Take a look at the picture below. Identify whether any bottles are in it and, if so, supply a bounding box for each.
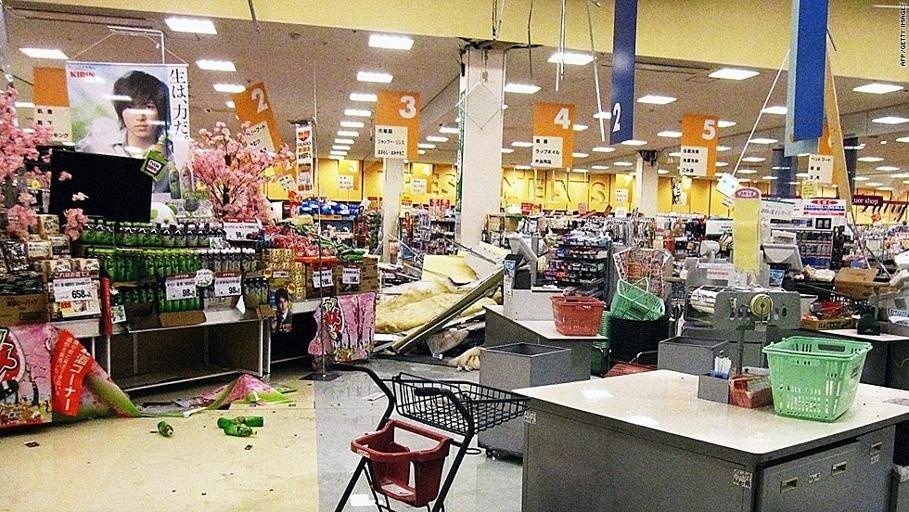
[157,421,174,436]
[216,416,264,437]
[168,161,180,199]
[179,161,193,195]
[74,217,269,315]
[144,134,167,161]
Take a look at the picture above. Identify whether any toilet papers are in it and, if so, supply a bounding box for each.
[697,354,732,402]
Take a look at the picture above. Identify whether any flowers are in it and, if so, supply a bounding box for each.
[0,54,94,250]
[179,116,296,224]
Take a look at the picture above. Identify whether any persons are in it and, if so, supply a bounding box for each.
[431,199,439,206]
[651,217,656,240]
[668,219,671,229]
[403,212,412,236]
[834,226,845,262]
[415,203,424,209]
[693,218,705,237]
[271,287,292,333]
[898,221,909,232]
[441,199,449,208]
[85,69,178,193]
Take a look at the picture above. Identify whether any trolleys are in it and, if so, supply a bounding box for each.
[321,358,524,512]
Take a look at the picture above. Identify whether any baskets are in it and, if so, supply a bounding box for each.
[588,275,679,379]
[548,295,606,337]
[760,334,874,425]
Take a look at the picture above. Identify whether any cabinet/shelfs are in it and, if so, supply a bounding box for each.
[400,201,909,310]
[0,200,381,423]
[507,364,908,511]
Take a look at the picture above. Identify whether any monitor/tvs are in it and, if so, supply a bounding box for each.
[48,150,156,224]
[762,243,804,272]
